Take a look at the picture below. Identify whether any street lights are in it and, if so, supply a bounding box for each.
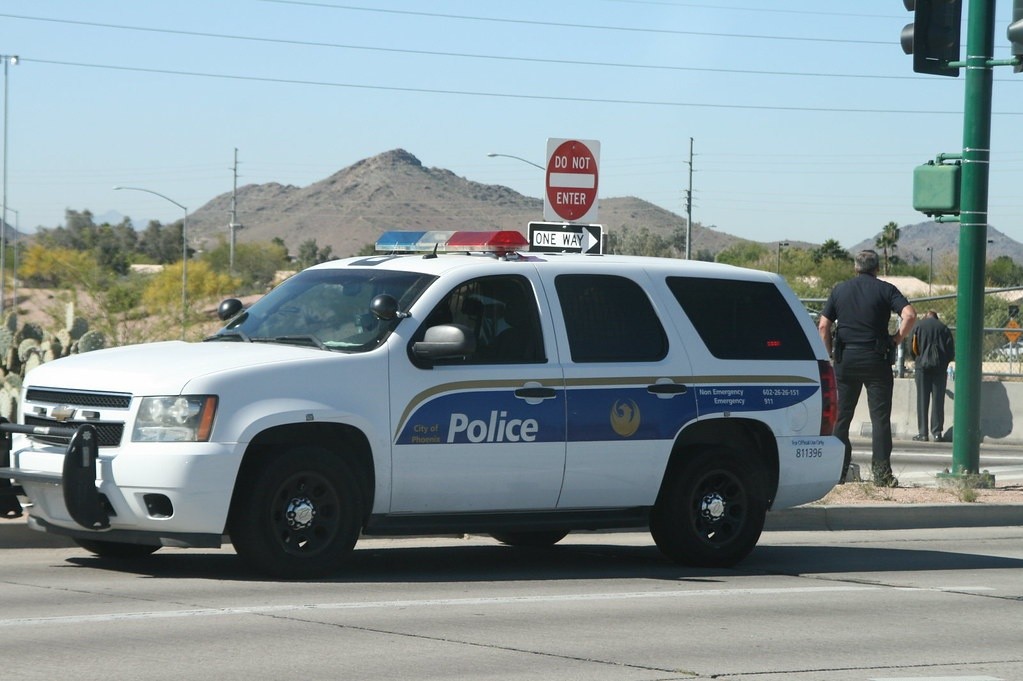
[924,247,933,298]
[113,186,187,341]
[777,241,789,276]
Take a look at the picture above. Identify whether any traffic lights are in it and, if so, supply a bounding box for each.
[542,137,601,225]
[900,0,961,77]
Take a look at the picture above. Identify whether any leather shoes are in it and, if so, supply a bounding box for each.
[934,434,944,442]
[912,434,927,441]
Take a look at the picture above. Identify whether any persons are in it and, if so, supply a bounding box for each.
[818,249,916,487]
[906,309,955,442]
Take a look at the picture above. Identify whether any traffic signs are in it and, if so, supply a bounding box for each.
[528,221,605,255]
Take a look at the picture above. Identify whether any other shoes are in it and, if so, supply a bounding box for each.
[875,474,899,487]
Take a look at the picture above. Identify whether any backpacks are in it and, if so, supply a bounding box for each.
[919,324,947,371]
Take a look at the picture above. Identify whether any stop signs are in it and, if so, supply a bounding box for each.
[7,229,852,585]
